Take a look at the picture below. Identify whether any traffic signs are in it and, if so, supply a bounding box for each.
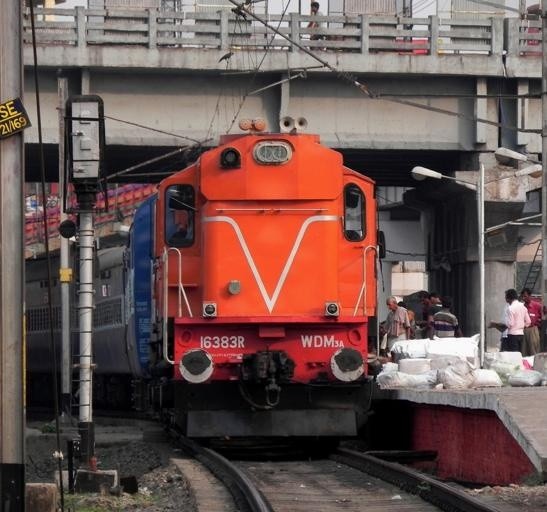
[411,164,487,370]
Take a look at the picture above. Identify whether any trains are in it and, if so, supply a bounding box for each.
[23,120,385,455]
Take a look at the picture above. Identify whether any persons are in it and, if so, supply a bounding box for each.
[378,291,464,364]
[487,288,531,355]
[518,288,542,357]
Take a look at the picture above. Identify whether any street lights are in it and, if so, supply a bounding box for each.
[493,144,544,171]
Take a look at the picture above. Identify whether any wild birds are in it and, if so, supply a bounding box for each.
[218,52,234,63]
[230,7,248,17]
